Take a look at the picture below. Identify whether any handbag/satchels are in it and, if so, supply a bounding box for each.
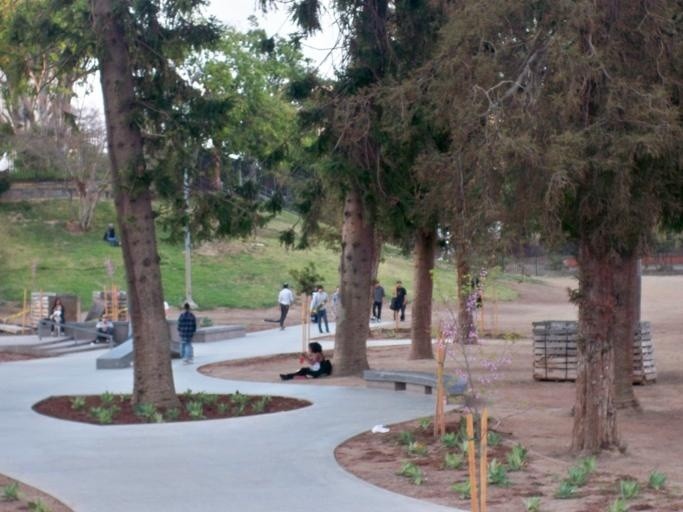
[311,312,318,323]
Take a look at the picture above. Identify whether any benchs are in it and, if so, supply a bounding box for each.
[362,368,451,393]
[194,325,247,344]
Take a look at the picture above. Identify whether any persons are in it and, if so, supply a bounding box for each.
[371,280,384,322]
[89,313,116,347]
[277,282,294,331]
[50,297,64,335]
[332,286,338,322]
[393,281,407,321]
[278,342,331,380]
[176,302,196,363]
[310,285,329,334]
[102,223,121,245]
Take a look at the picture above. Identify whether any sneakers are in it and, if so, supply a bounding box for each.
[182,360,194,365]
[279,373,293,380]
[371,316,406,322]
[280,325,329,333]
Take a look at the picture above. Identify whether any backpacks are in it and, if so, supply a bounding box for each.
[323,360,332,375]
